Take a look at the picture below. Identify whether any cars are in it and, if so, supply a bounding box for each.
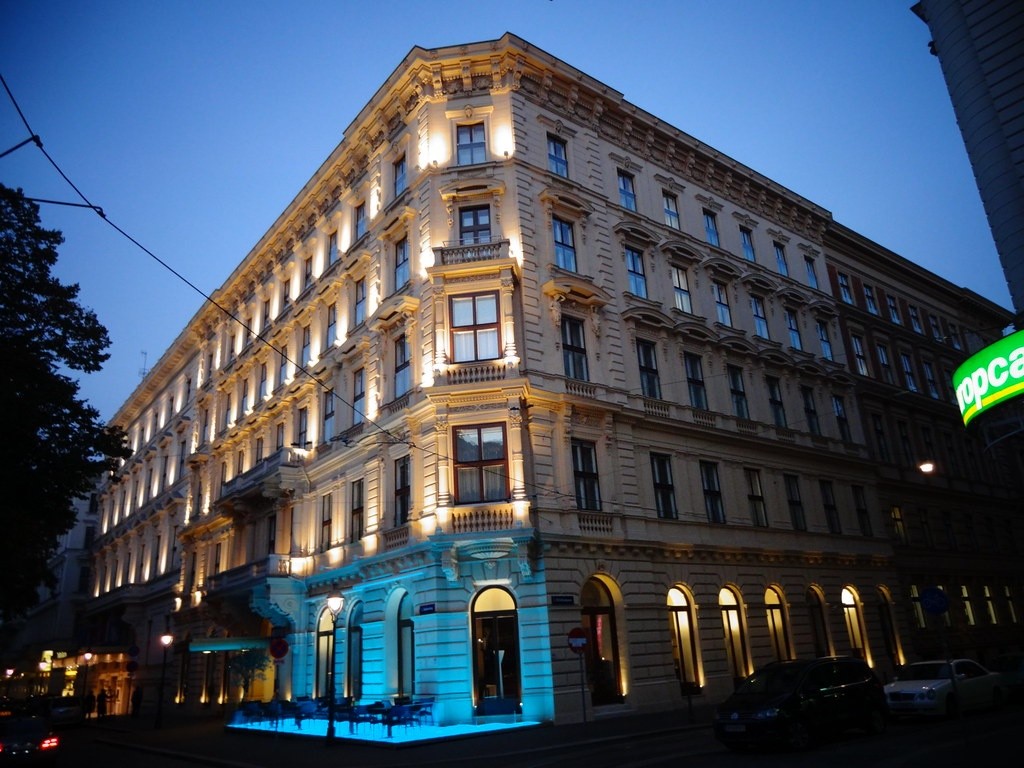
[0,713,65,768]
[711,656,889,756]
[883,656,1007,722]
[44,695,85,726]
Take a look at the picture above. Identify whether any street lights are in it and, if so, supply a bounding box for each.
[84,652,93,701]
[3,669,15,703]
[154,632,174,730]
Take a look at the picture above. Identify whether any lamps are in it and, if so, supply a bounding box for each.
[432,160,438,169]
[326,582,348,616]
[503,151,508,158]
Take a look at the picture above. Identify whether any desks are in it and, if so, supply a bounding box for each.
[371,708,388,734]
[403,703,432,726]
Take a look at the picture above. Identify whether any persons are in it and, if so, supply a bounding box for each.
[97,689,114,720]
[84,691,95,720]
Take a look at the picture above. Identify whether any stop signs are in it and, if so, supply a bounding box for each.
[567,626,588,655]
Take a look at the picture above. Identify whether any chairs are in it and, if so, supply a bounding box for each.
[235,693,437,738]
